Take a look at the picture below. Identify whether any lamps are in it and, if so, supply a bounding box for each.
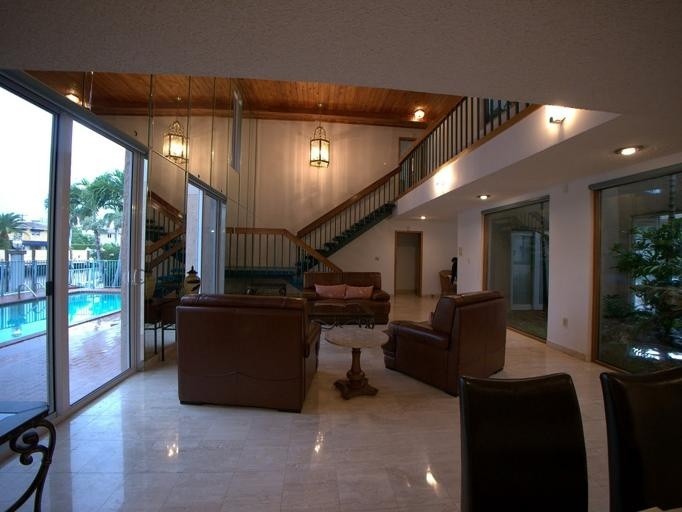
[162,96,190,166]
[308,103,331,168]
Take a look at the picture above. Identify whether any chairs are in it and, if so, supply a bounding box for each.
[455,372,592,511]
[382,288,508,398]
[175,292,324,414]
[598,364,681,509]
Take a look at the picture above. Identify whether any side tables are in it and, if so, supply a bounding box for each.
[308,304,389,400]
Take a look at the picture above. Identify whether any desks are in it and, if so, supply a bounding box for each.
[145,295,178,355]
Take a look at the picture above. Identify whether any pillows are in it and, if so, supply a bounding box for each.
[314,282,374,300]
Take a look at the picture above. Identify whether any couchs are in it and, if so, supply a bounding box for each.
[302,271,391,325]
